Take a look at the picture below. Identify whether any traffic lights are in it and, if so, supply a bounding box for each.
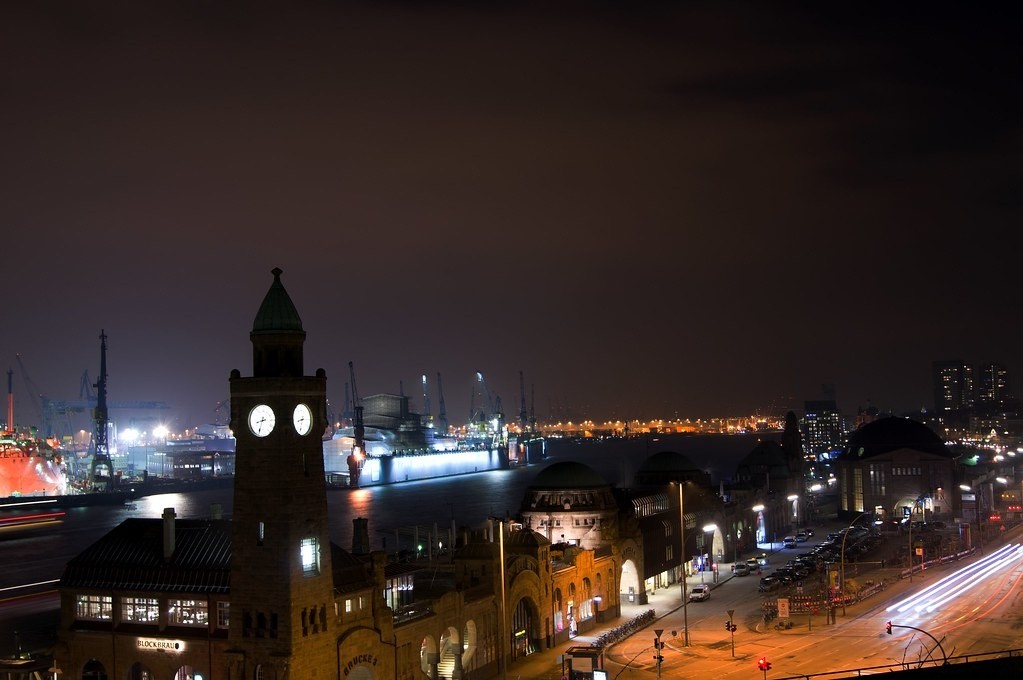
[766,661,772,671]
[654,638,657,648]
[653,656,656,659]
[659,656,664,662]
[758,665,763,671]
[660,642,664,650]
[886,620,892,634]
[732,624,737,632]
[882,559,886,568]
[724,621,731,631]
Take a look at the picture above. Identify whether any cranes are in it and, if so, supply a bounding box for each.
[340,359,538,445]
[13,326,172,494]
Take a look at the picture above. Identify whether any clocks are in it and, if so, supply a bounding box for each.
[247,404,276,438]
[292,402,314,438]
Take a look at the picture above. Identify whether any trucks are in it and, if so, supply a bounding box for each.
[689,585,712,602]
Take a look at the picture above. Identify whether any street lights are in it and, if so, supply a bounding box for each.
[907,486,943,582]
[841,511,873,617]
[759,657,767,680]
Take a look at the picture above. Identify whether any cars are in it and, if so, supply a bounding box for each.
[746,558,759,571]
[733,561,751,576]
[755,554,770,565]
[757,518,946,592]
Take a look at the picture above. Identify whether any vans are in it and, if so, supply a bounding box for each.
[783,536,797,549]
